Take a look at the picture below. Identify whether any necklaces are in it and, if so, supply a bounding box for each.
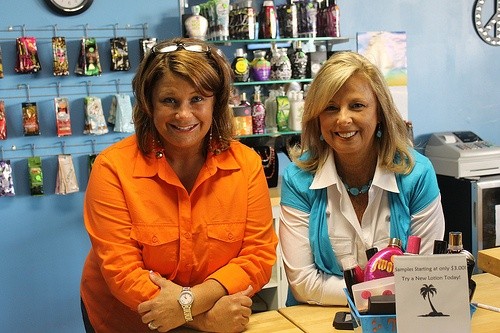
[340,177,372,196]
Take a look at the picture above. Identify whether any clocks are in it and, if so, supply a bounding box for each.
[43,0,94,15]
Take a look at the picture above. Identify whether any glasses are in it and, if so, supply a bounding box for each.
[140,40,221,80]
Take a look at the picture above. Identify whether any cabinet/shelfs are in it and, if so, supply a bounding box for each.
[180,0,352,138]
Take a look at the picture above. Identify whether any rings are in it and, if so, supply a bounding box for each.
[148,322,158,329]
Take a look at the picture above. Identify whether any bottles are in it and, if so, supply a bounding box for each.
[185,0,342,135]
[342,230,476,307]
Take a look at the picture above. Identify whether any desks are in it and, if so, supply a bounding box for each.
[238,273,500,333]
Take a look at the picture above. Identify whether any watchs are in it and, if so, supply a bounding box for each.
[178,286,194,323]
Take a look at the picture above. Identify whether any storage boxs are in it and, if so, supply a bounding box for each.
[341,285,477,333]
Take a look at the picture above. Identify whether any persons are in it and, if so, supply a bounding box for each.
[80,37,279,333]
[278,51,446,307]
